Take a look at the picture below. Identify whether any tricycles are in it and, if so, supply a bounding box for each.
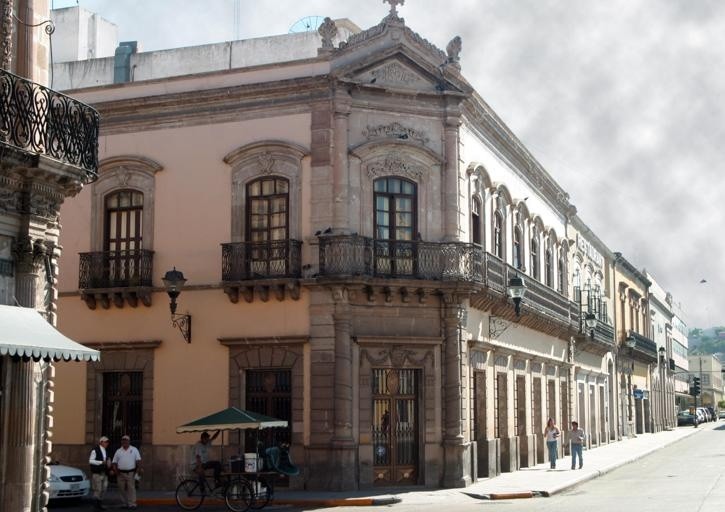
[175,406,289,512]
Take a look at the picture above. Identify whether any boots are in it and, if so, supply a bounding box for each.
[92,499,138,512]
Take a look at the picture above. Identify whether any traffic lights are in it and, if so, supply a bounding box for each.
[688,376,700,396]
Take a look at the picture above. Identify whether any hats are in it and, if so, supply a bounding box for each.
[122,435,131,440]
[100,436,109,442]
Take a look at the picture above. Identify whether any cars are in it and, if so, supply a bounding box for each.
[677,407,724,426]
[47,461,92,499]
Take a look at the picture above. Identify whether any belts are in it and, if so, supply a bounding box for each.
[119,468,136,473]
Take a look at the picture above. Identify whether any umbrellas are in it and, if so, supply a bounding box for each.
[176,406,288,474]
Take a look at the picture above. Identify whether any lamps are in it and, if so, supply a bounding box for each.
[488,273,528,339]
[658,347,665,362]
[625,332,636,352]
[585,310,598,341]
[162,267,192,344]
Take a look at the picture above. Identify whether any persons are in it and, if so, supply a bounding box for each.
[112,436,142,509]
[191,429,221,497]
[89,436,114,508]
[544,418,560,469]
[564,421,585,470]
